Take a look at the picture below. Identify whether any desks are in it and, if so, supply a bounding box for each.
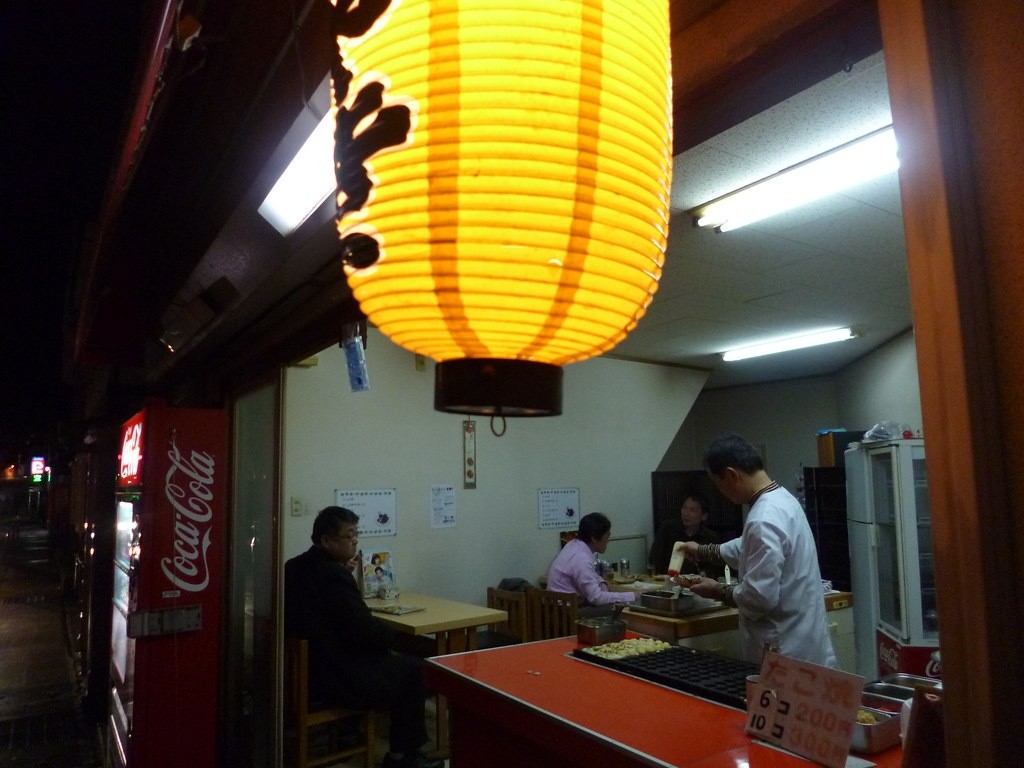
[608,573,669,594]
[364,591,509,761]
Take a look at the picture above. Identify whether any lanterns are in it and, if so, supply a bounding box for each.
[327,0,672,419]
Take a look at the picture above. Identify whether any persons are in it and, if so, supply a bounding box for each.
[282,505,437,768]
[645,488,723,584]
[679,433,839,672]
[545,511,636,619]
[364,552,396,598]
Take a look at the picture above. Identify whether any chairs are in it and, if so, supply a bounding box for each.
[256,615,376,768]
[476,586,579,650]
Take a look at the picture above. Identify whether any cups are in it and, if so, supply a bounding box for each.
[604,568,614,583]
[647,565,656,578]
[746,675,760,715]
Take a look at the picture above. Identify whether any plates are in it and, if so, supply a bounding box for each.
[613,579,635,584]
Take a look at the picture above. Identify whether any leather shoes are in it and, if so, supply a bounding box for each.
[381,751,442,768]
[337,732,365,763]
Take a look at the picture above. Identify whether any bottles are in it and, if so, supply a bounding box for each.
[667,541,686,577]
[718,576,738,586]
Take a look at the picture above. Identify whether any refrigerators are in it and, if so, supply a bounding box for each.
[105,407,230,768]
[867,439,942,679]
[844,447,878,685]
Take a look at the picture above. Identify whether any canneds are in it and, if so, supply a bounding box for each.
[621,559,630,576]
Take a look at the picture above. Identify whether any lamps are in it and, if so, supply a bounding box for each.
[720,324,865,362]
[688,125,898,234]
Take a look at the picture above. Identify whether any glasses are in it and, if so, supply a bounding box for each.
[338,532,359,540]
